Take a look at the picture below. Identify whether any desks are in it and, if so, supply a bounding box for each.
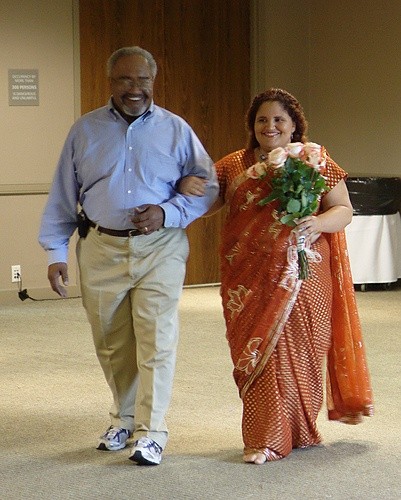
[344,212,401,291]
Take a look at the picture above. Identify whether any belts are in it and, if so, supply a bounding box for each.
[87,217,143,240]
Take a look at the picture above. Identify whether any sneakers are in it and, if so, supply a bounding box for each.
[95,425,133,452]
[127,437,162,465]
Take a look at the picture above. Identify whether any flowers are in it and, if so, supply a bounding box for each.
[244,142,330,293]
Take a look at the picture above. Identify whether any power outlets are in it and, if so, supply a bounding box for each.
[11,265,22,283]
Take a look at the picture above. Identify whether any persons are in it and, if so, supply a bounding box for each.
[178,89,374,464]
[39,47,219,464]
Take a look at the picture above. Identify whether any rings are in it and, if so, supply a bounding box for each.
[145,227,148,232]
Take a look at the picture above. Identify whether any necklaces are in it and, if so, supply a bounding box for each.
[257,147,269,162]
